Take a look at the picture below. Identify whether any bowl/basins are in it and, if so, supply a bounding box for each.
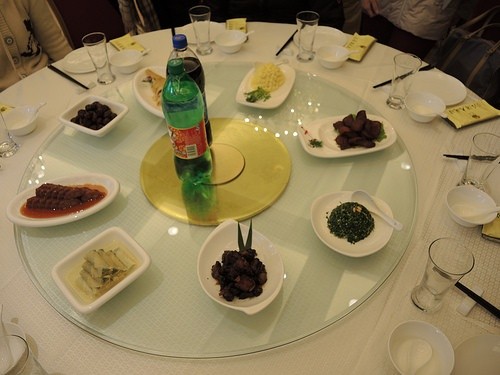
[309,191,394,258]
[51,226,152,315]
[0,20,500,375]
[58,93,129,137]
[387,320,455,374]
[196,218,284,316]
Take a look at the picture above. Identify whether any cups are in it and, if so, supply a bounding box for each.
[188,4,213,55]
[410,237,475,312]
[0,334,50,375]
[0,113,21,157]
[457,130,500,189]
[387,52,421,110]
[84,30,116,85]
[295,11,320,62]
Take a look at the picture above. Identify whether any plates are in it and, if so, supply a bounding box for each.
[297,115,397,158]
[0,317,27,375]
[132,65,178,120]
[235,62,297,109]
[6,175,120,227]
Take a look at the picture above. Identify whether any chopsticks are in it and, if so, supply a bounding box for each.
[432,266,500,322]
[431,266,500,319]
[172,27,178,57]
[443,154,496,161]
[276,23,305,56]
[373,64,434,88]
[47,65,90,90]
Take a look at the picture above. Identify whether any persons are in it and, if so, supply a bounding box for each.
[0,0,74,94]
[358,0,461,60]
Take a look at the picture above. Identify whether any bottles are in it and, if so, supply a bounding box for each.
[167,36,212,147]
[162,61,214,185]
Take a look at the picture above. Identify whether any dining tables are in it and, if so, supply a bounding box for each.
[0,22,500,375]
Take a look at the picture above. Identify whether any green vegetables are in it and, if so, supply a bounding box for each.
[326,202,375,243]
[242,87,270,103]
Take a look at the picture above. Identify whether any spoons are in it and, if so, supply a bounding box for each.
[403,338,432,375]
[456,205,500,219]
